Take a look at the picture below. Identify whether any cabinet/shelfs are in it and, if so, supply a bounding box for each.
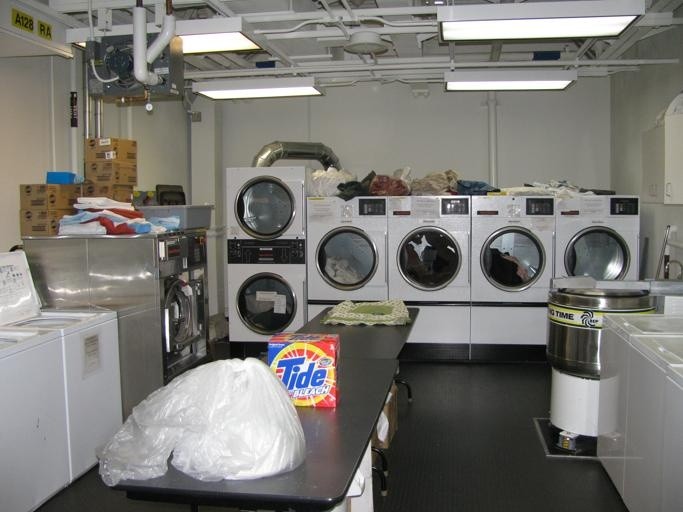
[642,112,683,205]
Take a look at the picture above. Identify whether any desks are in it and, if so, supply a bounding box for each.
[295,307,419,475]
[111,357,400,512]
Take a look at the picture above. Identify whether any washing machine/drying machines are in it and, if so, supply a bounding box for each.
[596,314,683,512]
[227,239,308,342]
[555,195,641,281]
[388,195,471,361]
[226,167,306,241]
[20,230,209,376]
[471,195,555,361]
[0,250,124,512]
[307,196,389,322]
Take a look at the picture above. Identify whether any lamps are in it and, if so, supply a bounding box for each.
[435,1,646,44]
[191,74,325,101]
[444,67,578,90]
[175,16,268,55]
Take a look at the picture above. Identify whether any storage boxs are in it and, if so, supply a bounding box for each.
[370,382,400,450]
[135,204,214,230]
[19,137,136,237]
